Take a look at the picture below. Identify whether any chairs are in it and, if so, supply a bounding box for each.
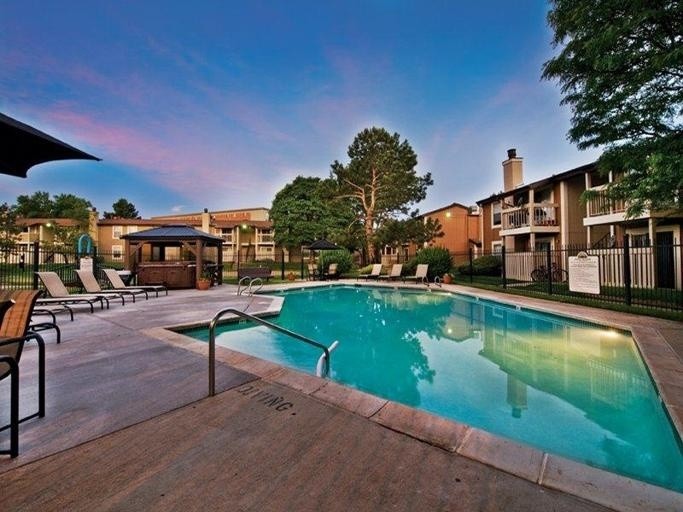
[401,263,429,284]
[377,264,403,283]
[0,261,169,461]
[356,263,382,282]
[306,263,321,280]
[323,262,341,281]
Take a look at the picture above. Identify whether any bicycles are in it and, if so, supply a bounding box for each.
[530,262,568,284]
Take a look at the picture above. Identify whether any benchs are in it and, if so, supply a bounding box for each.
[236,266,273,282]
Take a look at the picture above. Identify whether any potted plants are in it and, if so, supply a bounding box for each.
[196,272,213,290]
[442,271,454,285]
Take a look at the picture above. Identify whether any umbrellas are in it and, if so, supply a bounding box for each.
[0,102,104,181]
[302,239,343,272]
